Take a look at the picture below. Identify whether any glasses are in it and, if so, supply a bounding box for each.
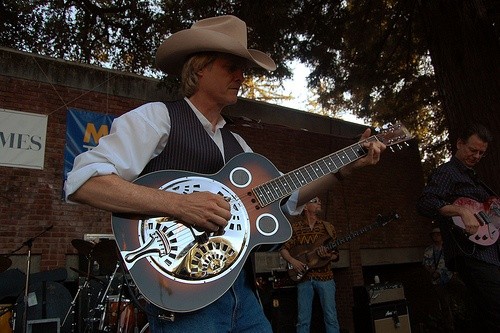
[309,198,322,203]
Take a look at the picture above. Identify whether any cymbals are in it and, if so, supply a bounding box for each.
[72,237,94,259]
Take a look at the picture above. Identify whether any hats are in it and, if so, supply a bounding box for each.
[155,15,277,72]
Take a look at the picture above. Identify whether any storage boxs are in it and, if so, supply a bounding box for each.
[368,284,407,305]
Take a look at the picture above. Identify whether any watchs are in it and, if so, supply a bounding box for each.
[331,168,345,182]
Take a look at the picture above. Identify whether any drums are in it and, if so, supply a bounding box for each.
[106,296,151,333]
[17,280,74,333]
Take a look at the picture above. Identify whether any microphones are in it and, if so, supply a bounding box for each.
[393,310,400,328]
[0,302,21,317]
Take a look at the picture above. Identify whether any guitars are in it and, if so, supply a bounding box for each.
[284,213,401,282]
[450,187,500,246]
[111,121,412,321]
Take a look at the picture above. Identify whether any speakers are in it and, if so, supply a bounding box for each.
[353,299,412,333]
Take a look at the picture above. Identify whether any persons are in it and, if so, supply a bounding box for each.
[278,196,340,333]
[63,15,387,333]
[416,123,500,333]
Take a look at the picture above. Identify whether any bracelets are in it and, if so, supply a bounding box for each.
[329,254,332,259]
